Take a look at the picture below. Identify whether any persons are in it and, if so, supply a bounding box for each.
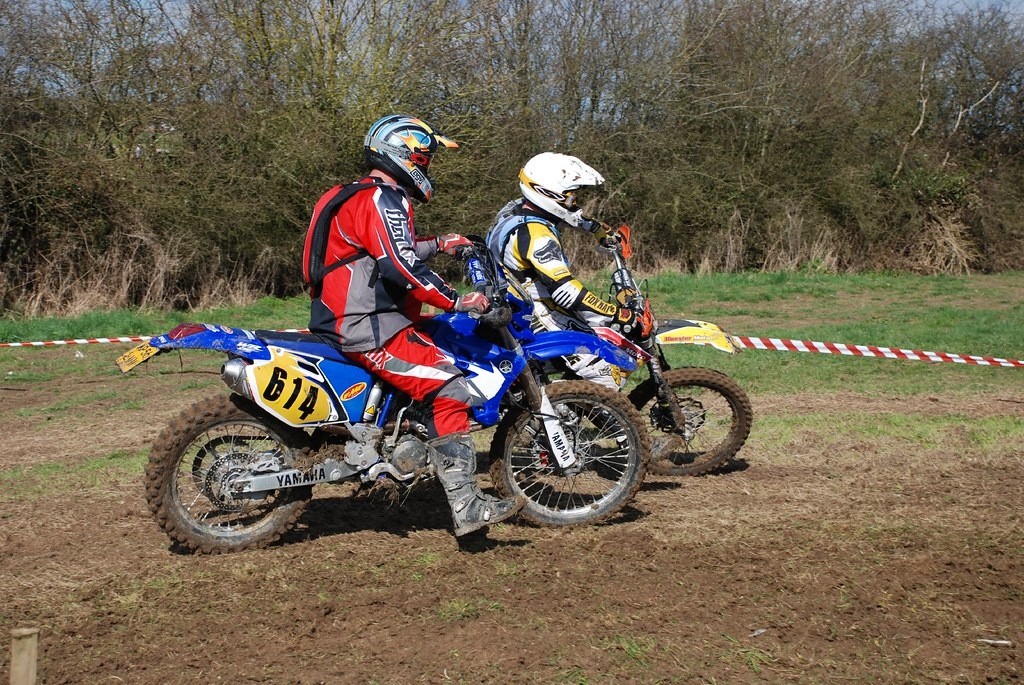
[302,115,525,541]
[484,152,654,474]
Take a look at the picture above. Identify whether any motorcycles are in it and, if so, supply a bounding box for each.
[498,223,754,481]
[114,235,651,556]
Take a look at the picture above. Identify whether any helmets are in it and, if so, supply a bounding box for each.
[517,151,605,228]
[364,114,459,203]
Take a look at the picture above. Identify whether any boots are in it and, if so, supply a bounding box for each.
[427,432,524,538]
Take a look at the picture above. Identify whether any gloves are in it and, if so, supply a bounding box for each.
[457,291,490,315]
[616,306,639,329]
[436,233,473,255]
[595,221,622,248]
